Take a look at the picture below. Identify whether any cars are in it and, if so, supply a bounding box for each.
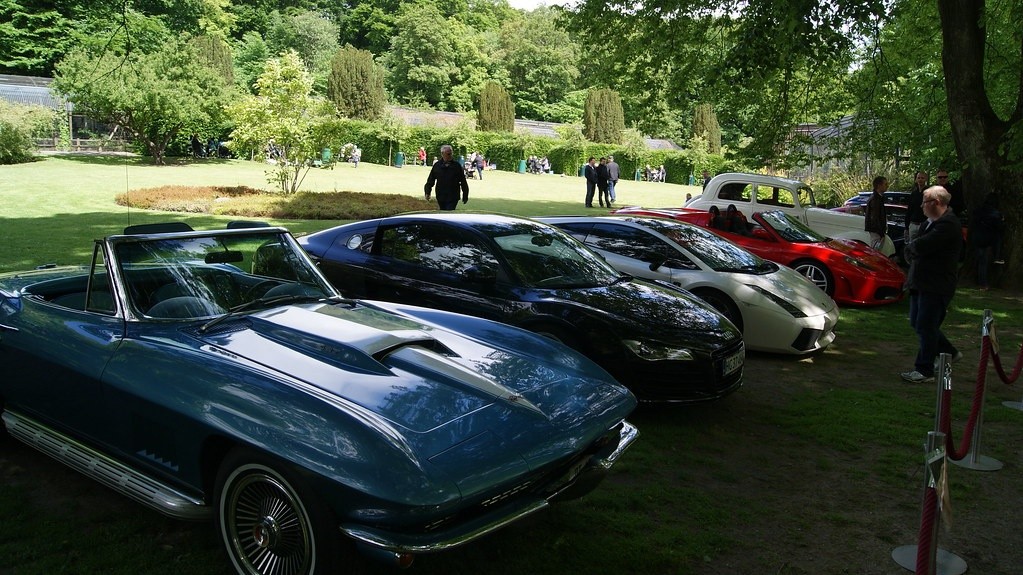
[829,191,913,266]
[682,172,897,263]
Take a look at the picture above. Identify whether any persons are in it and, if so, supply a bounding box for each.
[645,164,651,177]
[686,193,692,202]
[351,144,361,167]
[527,154,550,173]
[900,185,963,384]
[864,176,888,249]
[703,171,711,193]
[458,151,483,179]
[595,158,611,208]
[585,157,597,207]
[424,145,469,210]
[660,165,666,182]
[433,156,438,166]
[606,156,621,203]
[957,192,1003,290]
[936,170,962,216]
[419,147,427,166]
[190,134,215,159]
[904,171,929,230]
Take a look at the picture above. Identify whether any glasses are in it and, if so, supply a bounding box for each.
[937,176,948,179]
[917,177,924,179]
[922,198,939,204]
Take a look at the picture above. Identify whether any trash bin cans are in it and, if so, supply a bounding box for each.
[518,160,526,173]
[637,169,641,181]
[689,175,694,186]
[395,152,403,168]
[457,155,464,168]
[580,165,585,176]
[322,148,330,164]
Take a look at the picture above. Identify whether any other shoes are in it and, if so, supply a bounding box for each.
[586,203,611,208]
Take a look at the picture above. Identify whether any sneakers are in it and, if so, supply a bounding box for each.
[935,347,964,373]
[901,370,935,384]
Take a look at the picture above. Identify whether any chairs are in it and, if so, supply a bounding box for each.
[40,276,234,317]
[710,205,752,237]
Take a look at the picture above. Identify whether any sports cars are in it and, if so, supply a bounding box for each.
[490,213,840,360]
[0,221,644,575]
[251,209,747,421]
[610,202,909,310]
